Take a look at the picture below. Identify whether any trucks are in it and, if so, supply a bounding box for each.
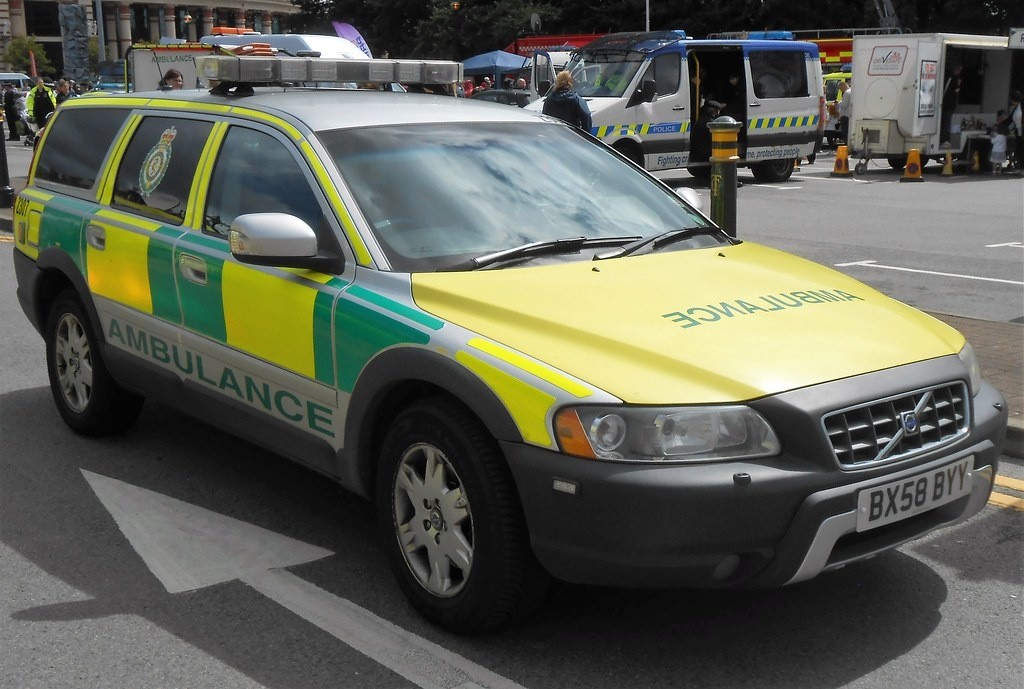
[845,27,1024,168]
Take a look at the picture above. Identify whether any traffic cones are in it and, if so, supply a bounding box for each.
[829,145,854,179]
[899,148,927,183]
[969,148,981,174]
[941,148,958,176]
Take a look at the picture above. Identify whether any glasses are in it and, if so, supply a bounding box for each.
[173,78,183,83]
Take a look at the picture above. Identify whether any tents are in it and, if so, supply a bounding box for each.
[461,49,532,75]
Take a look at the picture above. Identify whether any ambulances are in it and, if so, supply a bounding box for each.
[795,38,854,73]
[818,62,853,147]
[87,26,409,96]
[13,49,1011,644]
[517,31,829,180]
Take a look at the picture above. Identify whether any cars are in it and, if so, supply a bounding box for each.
[0,72,37,92]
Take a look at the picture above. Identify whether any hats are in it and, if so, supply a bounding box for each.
[707,101,726,109]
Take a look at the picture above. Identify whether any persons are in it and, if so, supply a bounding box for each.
[450,75,530,108]
[687,56,798,187]
[838,82,852,146]
[990,90,1024,176]
[814,85,839,157]
[356,81,448,96]
[157,69,184,91]
[380,49,389,59]
[542,70,592,133]
[796,153,816,165]
[941,73,963,148]
[0,78,95,194]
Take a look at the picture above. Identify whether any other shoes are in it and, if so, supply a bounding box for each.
[940,140,953,148]
[5,135,20,141]
[809,159,815,164]
[992,171,1001,177]
[830,151,837,155]
